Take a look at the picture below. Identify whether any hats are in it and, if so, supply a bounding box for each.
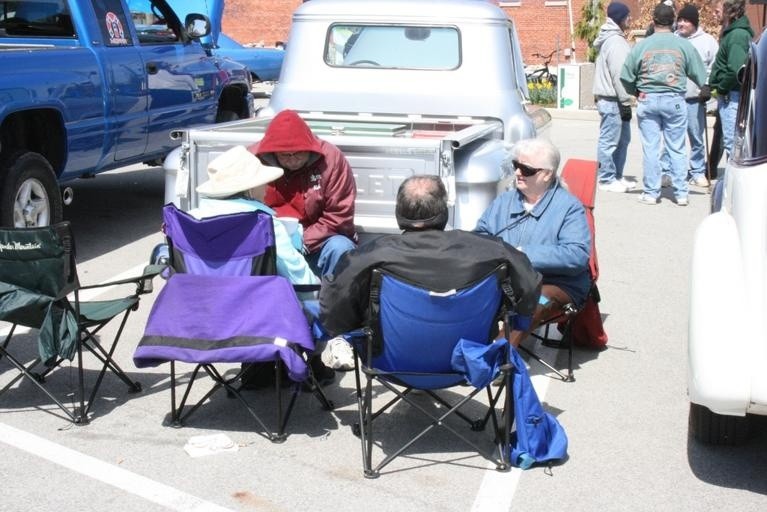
[676,5,699,31]
[649,0,675,26]
[195,145,285,198]
[607,1,630,24]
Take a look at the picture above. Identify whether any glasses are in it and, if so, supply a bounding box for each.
[512,159,553,176]
[276,151,309,161]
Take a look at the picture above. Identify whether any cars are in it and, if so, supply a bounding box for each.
[129,0,285,85]
[682,22,767,457]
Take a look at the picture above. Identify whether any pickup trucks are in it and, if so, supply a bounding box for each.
[0,0,259,235]
[158,1,553,244]
[38,84,152,150]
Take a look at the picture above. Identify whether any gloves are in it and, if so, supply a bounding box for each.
[716,86,730,95]
[619,104,632,121]
[699,84,713,103]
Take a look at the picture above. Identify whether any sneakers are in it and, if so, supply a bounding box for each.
[598,178,627,193]
[638,191,658,204]
[617,176,636,189]
[239,352,336,392]
[694,174,710,188]
[327,336,357,373]
[675,196,689,205]
[661,174,674,188]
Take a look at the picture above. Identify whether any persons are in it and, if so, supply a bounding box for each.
[620,4,708,206]
[170,143,335,392]
[255,109,357,298]
[658,5,720,189]
[646,1,679,37]
[593,1,635,186]
[708,0,754,162]
[318,175,542,357]
[473,138,593,386]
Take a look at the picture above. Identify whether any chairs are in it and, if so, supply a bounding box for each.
[0,220,164,426]
[518,158,601,382]
[341,262,521,479]
[162,202,335,442]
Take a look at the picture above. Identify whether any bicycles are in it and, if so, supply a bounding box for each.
[524,49,561,86]
